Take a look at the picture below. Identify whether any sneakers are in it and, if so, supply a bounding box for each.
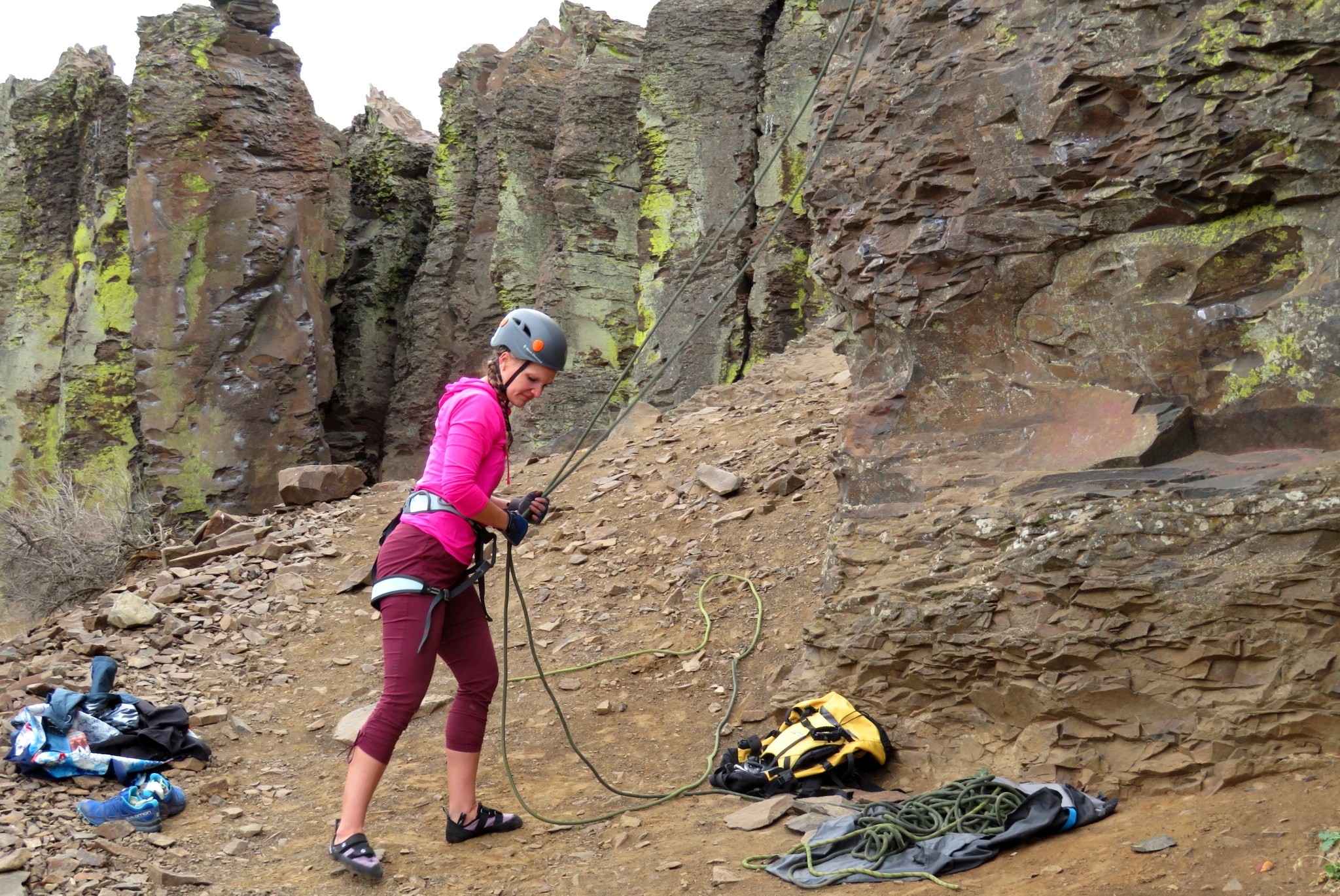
[77,785,162,834]
[138,773,185,818]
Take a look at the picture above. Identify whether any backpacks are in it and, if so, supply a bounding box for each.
[708,690,899,803]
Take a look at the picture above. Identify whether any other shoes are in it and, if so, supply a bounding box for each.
[329,819,384,879]
[442,803,522,842]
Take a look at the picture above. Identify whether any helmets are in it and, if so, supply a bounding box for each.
[490,308,568,371]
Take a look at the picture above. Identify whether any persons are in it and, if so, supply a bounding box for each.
[330,309,567,881]
